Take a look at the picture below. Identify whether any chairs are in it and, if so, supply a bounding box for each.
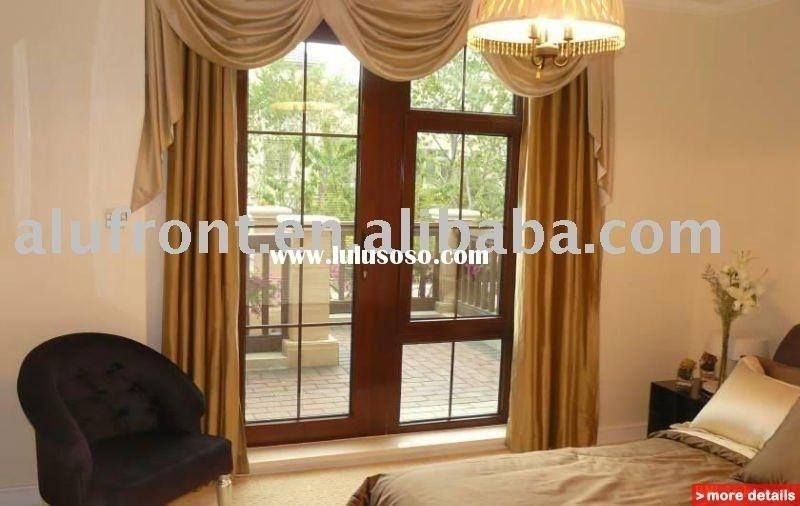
[17,333,234,506]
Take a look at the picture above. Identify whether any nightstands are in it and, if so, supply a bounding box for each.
[647,378,727,435]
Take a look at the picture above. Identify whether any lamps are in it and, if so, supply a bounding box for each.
[466,0,626,81]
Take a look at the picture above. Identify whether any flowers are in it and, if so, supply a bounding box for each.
[701,248,769,335]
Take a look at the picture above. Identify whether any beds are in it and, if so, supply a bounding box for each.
[346,323,799,506]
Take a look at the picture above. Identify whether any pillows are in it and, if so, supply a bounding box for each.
[651,354,800,484]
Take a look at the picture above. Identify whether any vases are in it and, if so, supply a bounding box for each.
[678,357,697,382]
[718,336,729,389]
[699,351,718,380]
